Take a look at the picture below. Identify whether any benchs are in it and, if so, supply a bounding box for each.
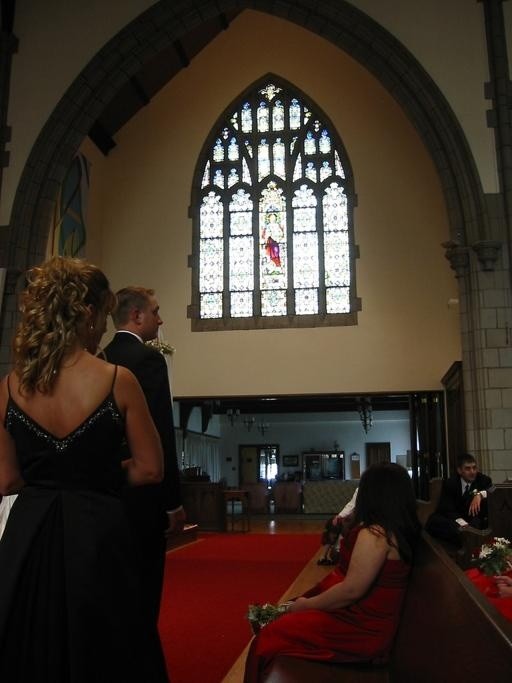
[251,527,511,683]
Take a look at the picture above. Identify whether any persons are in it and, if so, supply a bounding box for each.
[241,459,429,681]
[95,284,186,626]
[261,213,283,272]
[1,254,169,683]
[315,481,361,567]
[422,451,495,549]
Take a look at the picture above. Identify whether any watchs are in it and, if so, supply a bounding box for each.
[476,493,483,499]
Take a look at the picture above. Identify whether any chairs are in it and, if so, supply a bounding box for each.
[242,479,358,515]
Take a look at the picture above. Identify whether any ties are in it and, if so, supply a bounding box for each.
[461,483,470,496]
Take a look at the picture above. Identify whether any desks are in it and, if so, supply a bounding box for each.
[221,487,252,534]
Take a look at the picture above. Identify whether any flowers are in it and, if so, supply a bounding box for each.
[246,599,288,636]
[467,536,511,579]
